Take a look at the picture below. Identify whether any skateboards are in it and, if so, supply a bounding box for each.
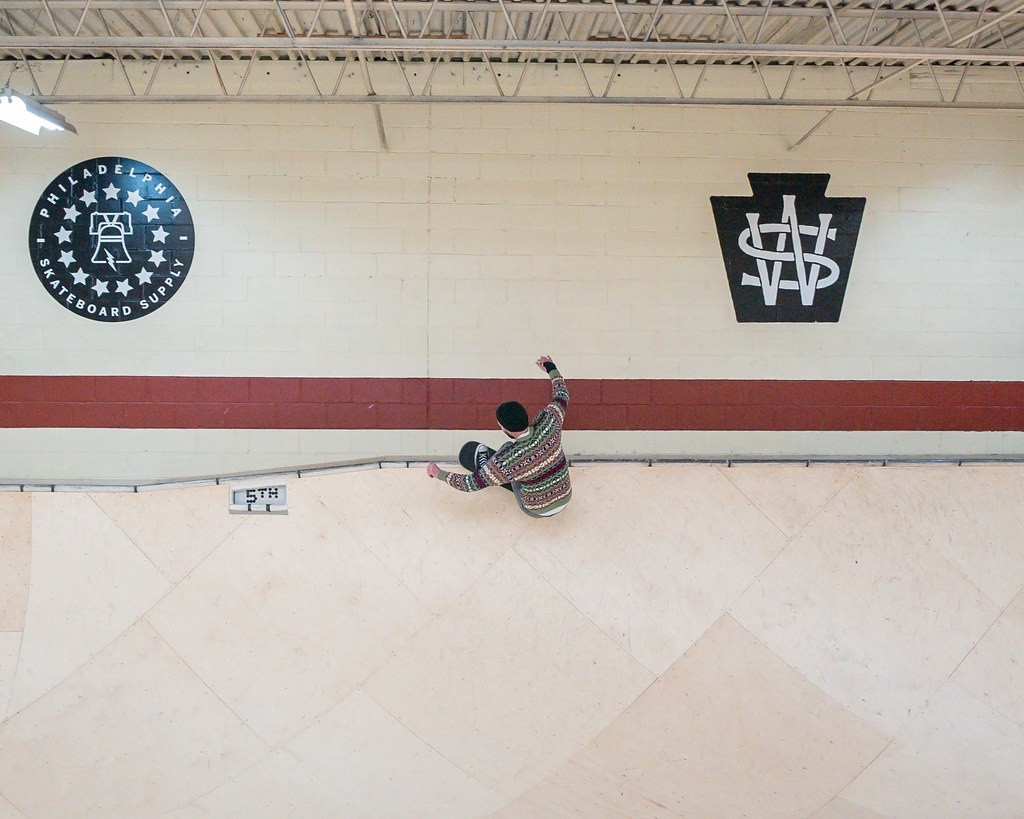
[457,440,514,493]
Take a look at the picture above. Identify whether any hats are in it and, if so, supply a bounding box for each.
[496,401,530,431]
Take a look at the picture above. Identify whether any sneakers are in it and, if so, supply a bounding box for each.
[474,445,490,469]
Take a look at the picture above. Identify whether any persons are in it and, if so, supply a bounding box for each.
[428,355,572,518]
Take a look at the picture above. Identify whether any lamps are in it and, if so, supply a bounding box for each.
[0,6,79,136]
